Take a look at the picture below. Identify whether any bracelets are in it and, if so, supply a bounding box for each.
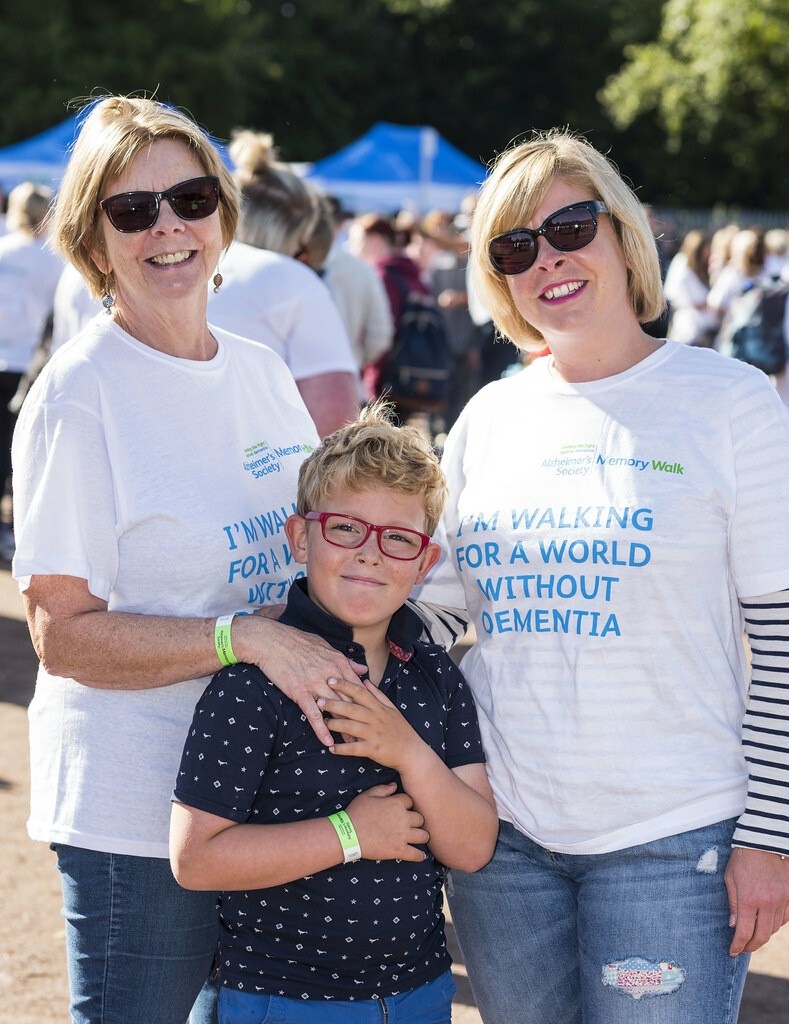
[329,808,364,865]
[215,609,246,668]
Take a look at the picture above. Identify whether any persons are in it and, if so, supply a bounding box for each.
[245,138,789,1023]
[48,219,363,446]
[0,179,63,525]
[12,98,390,1023]
[168,417,500,1024]
[212,132,787,459]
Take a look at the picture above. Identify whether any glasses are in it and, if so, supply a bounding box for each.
[485,199,610,275]
[93,175,222,234]
[305,509,434,562]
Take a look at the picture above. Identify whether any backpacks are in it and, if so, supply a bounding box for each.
[372,264,456,415]
[732,284,789,375]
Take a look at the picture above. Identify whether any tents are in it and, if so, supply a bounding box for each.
[0,92,265,212]
[284,123,511,227]
